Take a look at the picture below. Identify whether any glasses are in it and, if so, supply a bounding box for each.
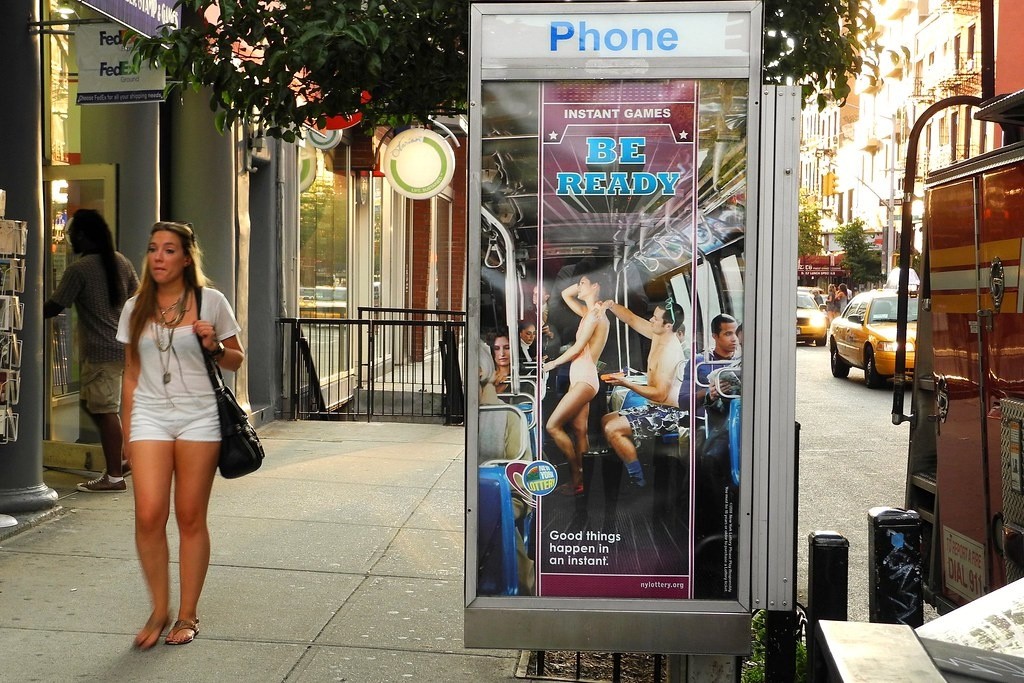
[65,229,75,236]
[175,221,197,248]
[666,296,676,326]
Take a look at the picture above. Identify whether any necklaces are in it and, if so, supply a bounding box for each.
[151,291,193,382]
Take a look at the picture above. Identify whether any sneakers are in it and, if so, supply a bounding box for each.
[76,468,127,492]
[122,460,132,477]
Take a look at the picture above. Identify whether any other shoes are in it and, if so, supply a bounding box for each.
[554,483,584,495]
[612,478,647,499]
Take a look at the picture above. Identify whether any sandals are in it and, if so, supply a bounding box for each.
[134,611,171,648]
[165,617,200,644]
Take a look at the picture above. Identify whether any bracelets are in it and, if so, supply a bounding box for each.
[609,300,614,309]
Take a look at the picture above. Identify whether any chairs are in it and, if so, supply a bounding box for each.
[476,359,741,594]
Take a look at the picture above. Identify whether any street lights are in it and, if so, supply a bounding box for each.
[820,93,895,278]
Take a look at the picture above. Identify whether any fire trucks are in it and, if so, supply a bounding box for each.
[892,90,1024,618]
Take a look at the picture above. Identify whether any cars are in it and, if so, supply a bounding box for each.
[796,291,828,346]
[828,289,919,389]
[797,286,824,296]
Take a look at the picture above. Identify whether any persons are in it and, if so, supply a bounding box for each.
[605,258,650,372]
[43,209,140,492]
[543,271,610,496]
[835,283,848,314]
[678,313,743,473]
[115,221,246,650]
[548,257,597,309]
[532,282,561,363]
[812,289,824,306]
[596,299,691,500]
[478,339,533,520]
[519,321,537,362]
[486,324,510,400]
[826,283,835,322]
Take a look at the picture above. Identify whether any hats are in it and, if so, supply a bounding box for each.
[812,289,818,293]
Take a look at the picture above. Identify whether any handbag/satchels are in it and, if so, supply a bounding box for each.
[194,285,265,479]
